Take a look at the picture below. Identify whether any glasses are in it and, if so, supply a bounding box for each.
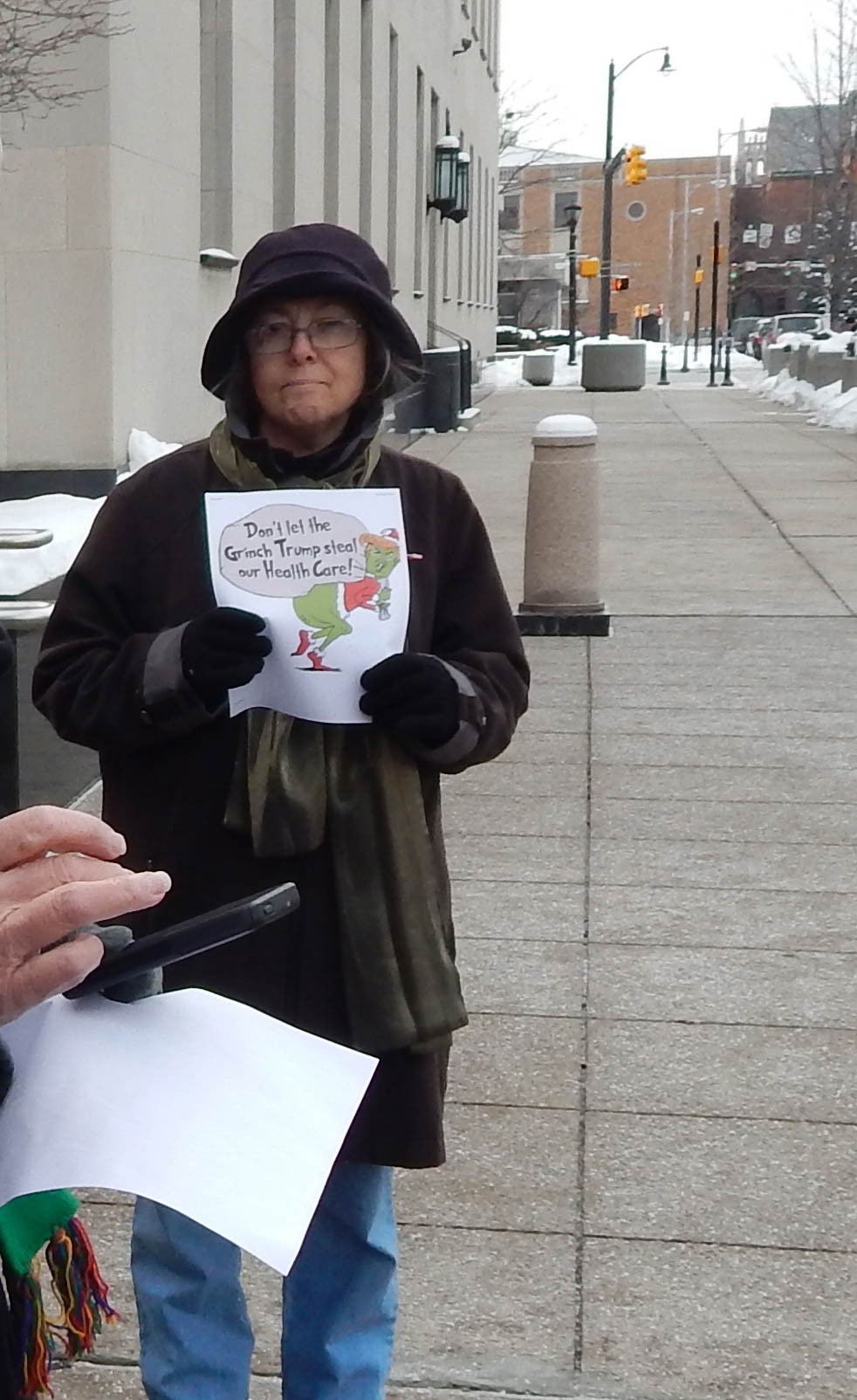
[243,315,362,357]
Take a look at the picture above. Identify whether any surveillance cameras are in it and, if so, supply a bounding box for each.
[460,38,471,52]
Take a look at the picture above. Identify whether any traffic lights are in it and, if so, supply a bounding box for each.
[615,276,629,290]
[694,270,704,285]
[623,148,647,187]
[712,243,726,266]
[578,257,599,276]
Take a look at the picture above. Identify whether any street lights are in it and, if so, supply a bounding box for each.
[599,45,672,341]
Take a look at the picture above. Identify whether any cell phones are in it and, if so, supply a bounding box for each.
[58,880,302,1000]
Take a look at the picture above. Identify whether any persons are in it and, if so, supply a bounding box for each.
[2,807,174,1399]
[33,228,530,1400]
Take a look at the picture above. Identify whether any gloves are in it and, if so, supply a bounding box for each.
[361,650,460,753]
[181,606,272,704]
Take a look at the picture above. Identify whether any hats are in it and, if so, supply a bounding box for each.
[201,224,423,403]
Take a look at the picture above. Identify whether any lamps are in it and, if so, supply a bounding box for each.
[440,152,470,226]
[426,135,461,217]
